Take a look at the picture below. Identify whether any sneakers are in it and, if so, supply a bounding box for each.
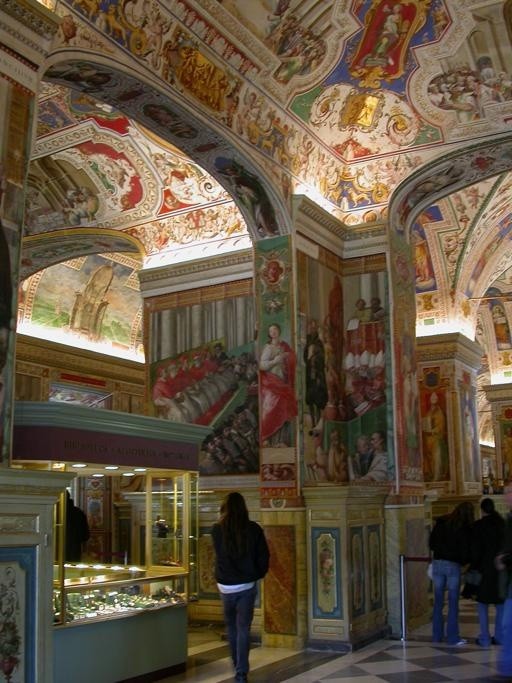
[234,671,247,683]
[456,638,468,645]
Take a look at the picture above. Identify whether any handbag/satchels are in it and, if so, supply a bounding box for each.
[463,564,483,586]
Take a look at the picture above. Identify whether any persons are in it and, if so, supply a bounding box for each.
[208,489,271,681]
[428,500,475,646]
[460,496,509,647]
[493,506,512,681]
[63,486,93,562]
